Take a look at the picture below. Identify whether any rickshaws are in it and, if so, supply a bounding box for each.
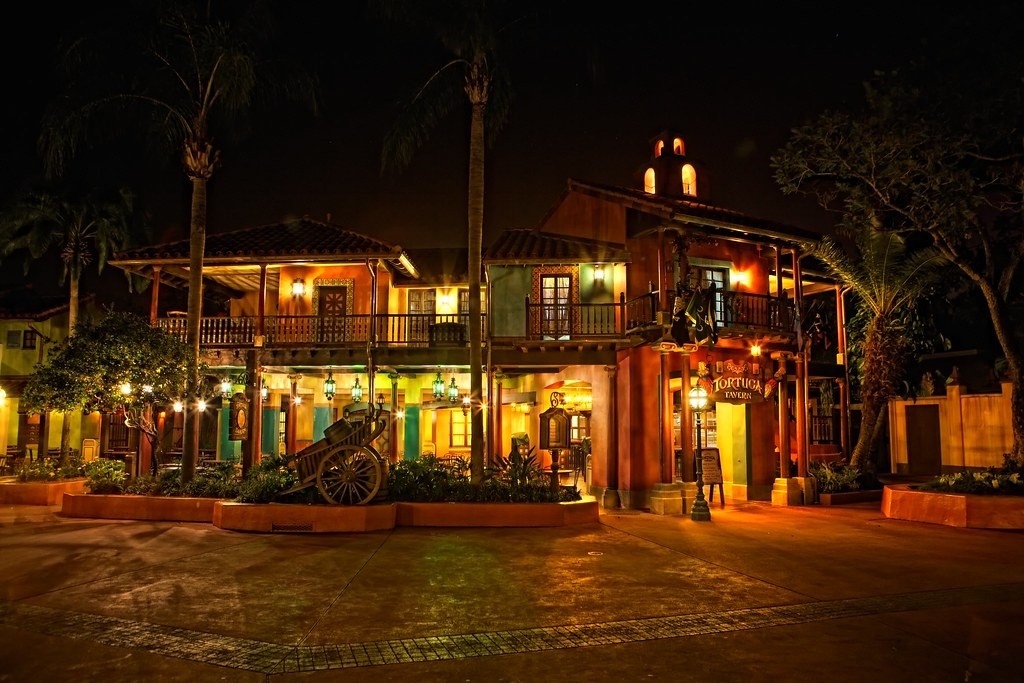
[271,400,388,505]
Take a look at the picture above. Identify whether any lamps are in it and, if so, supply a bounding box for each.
[323,372,337,400]
[351,378,362,403]
[377,392,385,409]
[433,372,458,404]
[292,277,304,300]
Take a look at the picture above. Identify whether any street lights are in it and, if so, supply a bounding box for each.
[687,381,712,521]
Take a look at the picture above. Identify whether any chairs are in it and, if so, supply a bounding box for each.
[1,447,244,479]
